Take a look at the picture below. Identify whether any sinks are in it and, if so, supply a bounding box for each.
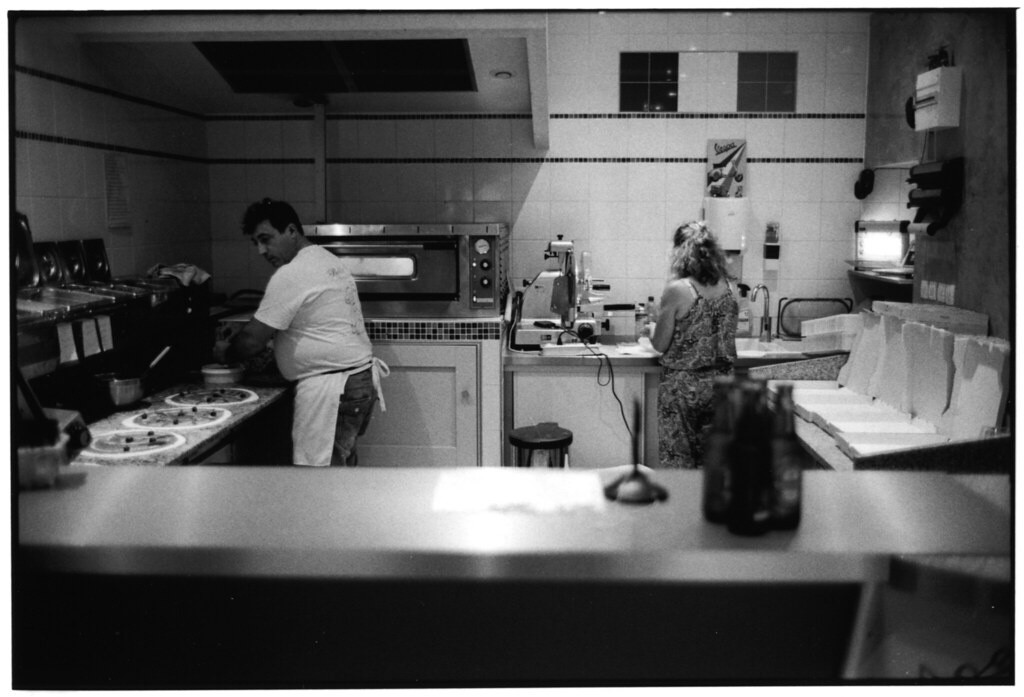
[735,340,766,353]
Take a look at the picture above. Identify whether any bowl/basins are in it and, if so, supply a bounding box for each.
[94,372,143,407]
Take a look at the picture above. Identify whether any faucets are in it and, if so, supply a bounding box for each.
[751,283,772,342]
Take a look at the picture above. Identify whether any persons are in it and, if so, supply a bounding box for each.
[642,221,739,469]
[213,197,378,468]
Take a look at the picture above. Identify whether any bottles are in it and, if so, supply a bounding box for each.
[702,370,802,537]
[647,296,656,323]
[635,303,647,341]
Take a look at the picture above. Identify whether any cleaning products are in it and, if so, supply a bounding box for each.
[734,283,753,338]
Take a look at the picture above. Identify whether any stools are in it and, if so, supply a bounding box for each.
[508,425,573,467]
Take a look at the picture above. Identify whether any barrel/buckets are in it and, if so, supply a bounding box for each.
[201,363,246,384]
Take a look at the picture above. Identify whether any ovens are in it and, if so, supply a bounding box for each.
[316,237,498,319]
